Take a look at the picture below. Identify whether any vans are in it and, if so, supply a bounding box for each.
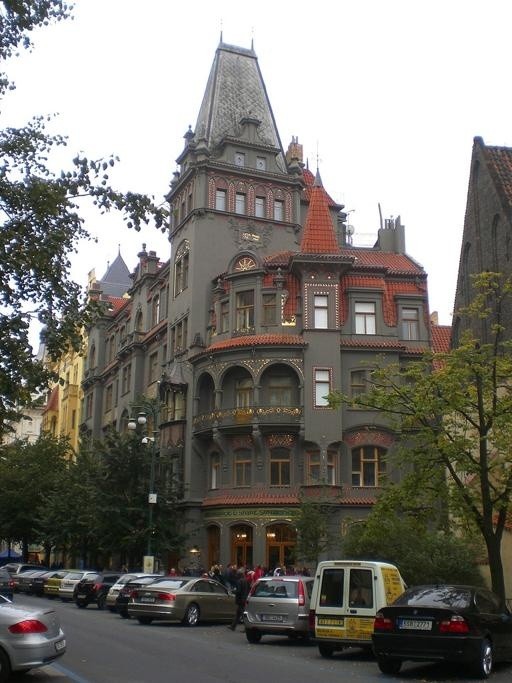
[307,559,406,658]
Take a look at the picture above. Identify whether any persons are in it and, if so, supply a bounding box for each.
[228,568,250,632]
[202,562,311,586]
[169,568,177,577]
[51,562,64,569]
[122,562,128,574]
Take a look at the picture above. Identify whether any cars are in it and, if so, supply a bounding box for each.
[0,563,163,619]
[372,584,511,679]
[0,593,67,678]
[242,573,315,644]
[127,574,237,628]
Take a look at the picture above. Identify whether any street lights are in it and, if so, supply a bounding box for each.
[126,401,159,573]
